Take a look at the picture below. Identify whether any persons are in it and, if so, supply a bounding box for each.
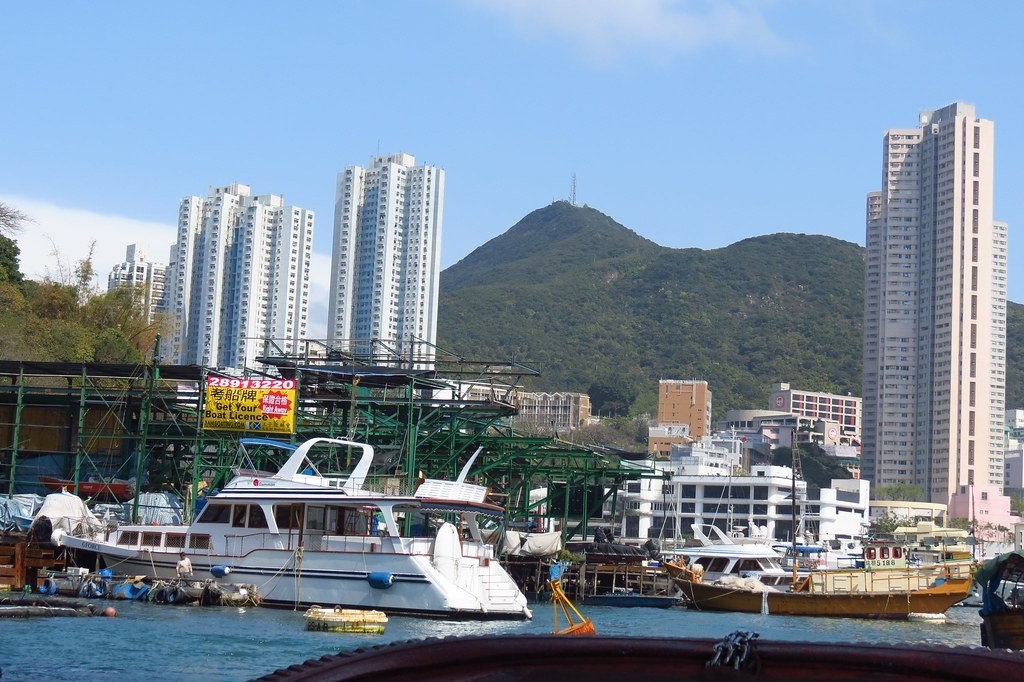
[176,552,193,587]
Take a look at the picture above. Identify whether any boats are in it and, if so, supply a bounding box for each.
[17,337,977,621]
[303,604,389,636]
[963,550,1024,649]
